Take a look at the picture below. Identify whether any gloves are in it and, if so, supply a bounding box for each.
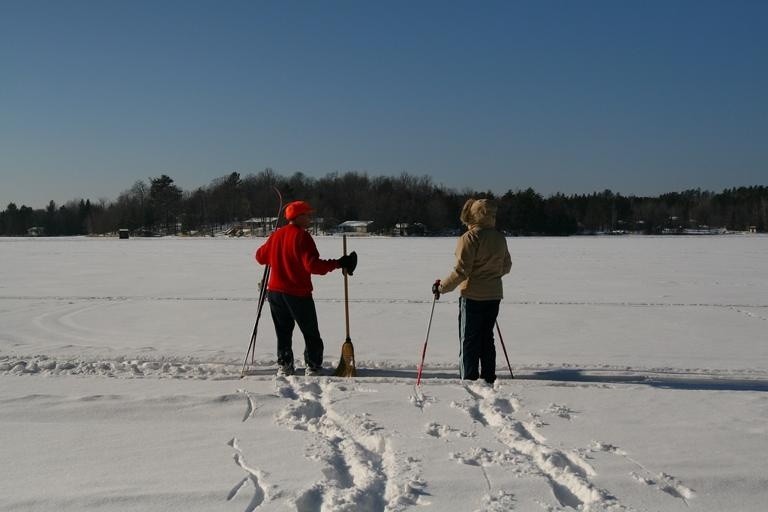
[339,255,353,267]
[432,283,440,294]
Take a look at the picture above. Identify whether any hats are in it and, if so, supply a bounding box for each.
[284,200,318,220]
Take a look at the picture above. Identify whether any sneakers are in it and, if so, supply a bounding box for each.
[276,364,294,377]
[304,366,334,376]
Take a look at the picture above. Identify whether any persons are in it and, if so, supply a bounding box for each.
[432,199,512,383]
[255,200,358,376]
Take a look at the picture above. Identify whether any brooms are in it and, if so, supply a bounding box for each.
[334,234,357,378]
